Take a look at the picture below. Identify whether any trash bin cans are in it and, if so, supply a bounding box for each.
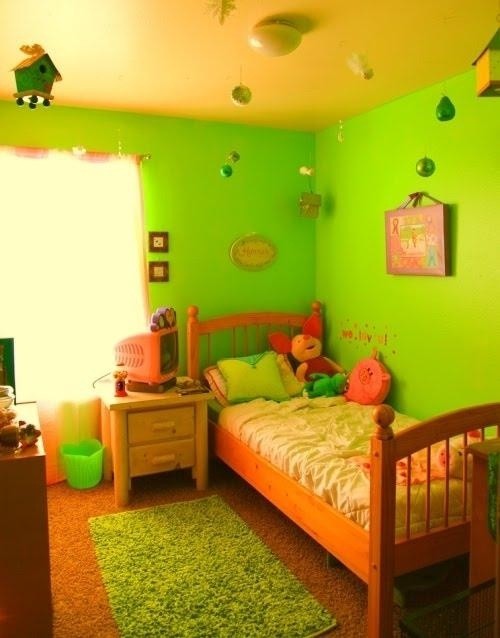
[59,438,103,489]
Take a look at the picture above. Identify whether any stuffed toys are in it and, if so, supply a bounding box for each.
[343,346,392,406]
[302,370,348,399]
[266,311,344,382]
[358,429,486,487]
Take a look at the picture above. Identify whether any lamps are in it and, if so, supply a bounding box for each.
[246,19,302,58]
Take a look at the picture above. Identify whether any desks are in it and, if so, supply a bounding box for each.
[0,403,55,638]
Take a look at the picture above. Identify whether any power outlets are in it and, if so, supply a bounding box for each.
[149,232,168,252]
[149,261,169,282]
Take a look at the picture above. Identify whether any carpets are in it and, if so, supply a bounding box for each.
[88,494,338,638]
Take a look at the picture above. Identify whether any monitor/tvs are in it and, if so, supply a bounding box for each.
[112,326,178,384]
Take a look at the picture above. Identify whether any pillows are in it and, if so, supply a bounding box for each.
[201,354,300,408]
[217,350,289,402]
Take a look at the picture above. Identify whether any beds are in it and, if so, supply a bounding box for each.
[187,300,500,638]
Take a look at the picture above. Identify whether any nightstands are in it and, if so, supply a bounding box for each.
[96,377,216,507]
